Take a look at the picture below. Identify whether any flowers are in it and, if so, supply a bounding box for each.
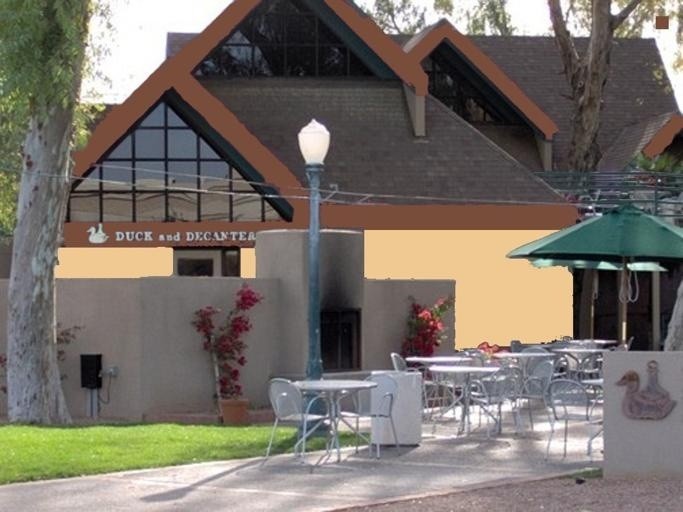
[193,279,263,399]
[401,292,456,366]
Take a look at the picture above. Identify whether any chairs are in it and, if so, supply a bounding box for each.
[540,377,606,463]
[265,378,333,464]
[334,372,402,462]
[390,339,632,423]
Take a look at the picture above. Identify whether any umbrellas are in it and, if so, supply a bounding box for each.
[527,198,681,344]
[503,216,672,344]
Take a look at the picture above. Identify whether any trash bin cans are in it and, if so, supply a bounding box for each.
[370,370,424,445]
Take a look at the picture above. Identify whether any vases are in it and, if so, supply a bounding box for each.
[220,399,249,426]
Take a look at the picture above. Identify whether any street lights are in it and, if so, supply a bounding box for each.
[296,115,334,443]
